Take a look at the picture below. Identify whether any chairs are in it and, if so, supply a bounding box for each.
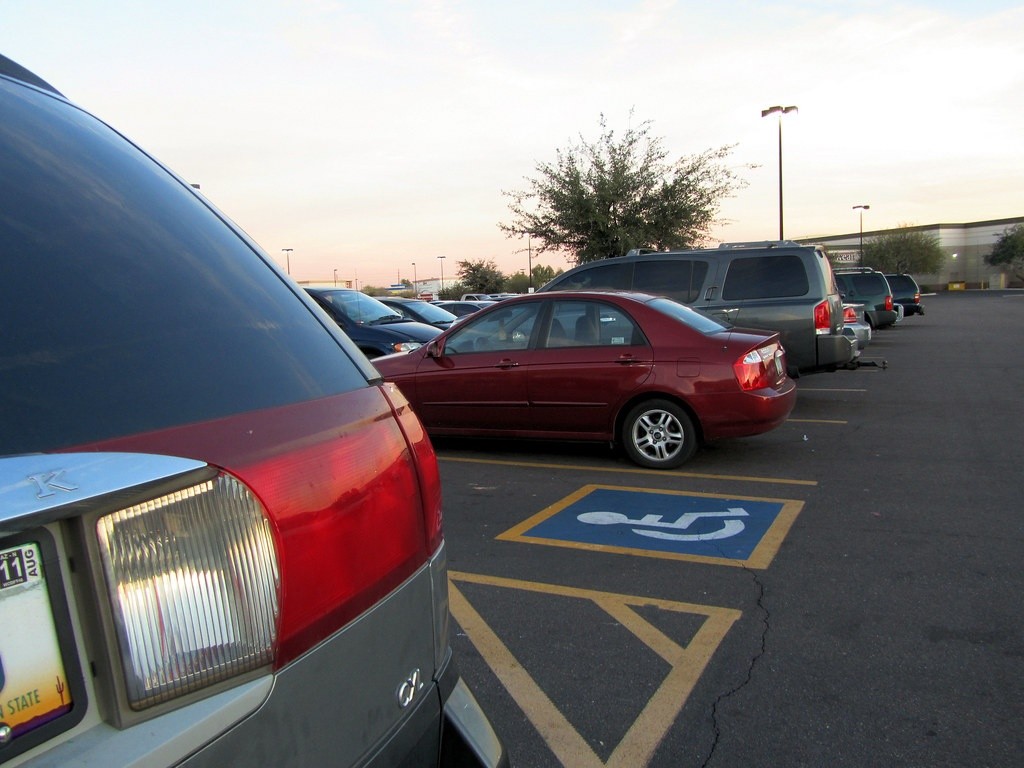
[574,315,599,344]
[550,319,568,339]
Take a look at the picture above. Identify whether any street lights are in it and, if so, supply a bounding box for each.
[851,204,870,267]
[333,269,338,287]
[522,232,536,287]
[411,262,418,300]
[760,105,799,241]
[281,248,294,275]
[355,278,359,290]
[436,256,447,291]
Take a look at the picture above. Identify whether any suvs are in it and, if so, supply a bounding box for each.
[303,285,446,360]
[883,270,926,318]
[488,239,890,381]
[0,51,515,768]
[833,265,904,330]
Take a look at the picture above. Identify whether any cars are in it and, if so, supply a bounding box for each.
[364,287,797,471]
[372,292,525,333]
